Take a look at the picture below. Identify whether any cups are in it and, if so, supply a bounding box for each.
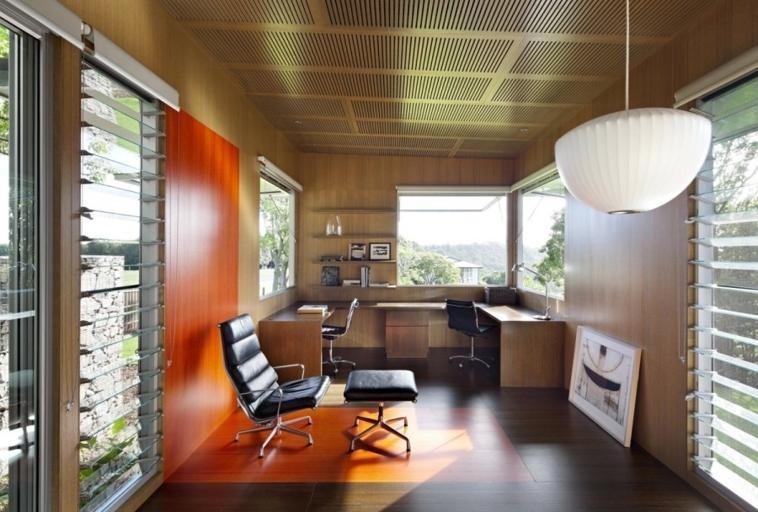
[326,215,343,236]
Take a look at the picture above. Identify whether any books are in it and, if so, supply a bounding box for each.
[360,266,369,287]
[298,304,329,314]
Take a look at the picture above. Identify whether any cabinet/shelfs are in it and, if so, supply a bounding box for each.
[384,312,430,359]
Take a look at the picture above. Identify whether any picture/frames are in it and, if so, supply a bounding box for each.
[567,325,642,448]
[348,242,391,261]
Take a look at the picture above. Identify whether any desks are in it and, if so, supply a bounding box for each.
[479,303,564,385]
[334,303,478,311]
[259,302,333,382]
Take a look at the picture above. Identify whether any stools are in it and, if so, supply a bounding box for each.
[342,368,418,454]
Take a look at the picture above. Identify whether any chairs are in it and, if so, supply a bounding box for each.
[217,311,332,458]
[447,299,498,368]
[322,297,358,378]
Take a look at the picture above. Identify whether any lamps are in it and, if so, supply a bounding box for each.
[511,265,552,319]
[554,0,713,215]
